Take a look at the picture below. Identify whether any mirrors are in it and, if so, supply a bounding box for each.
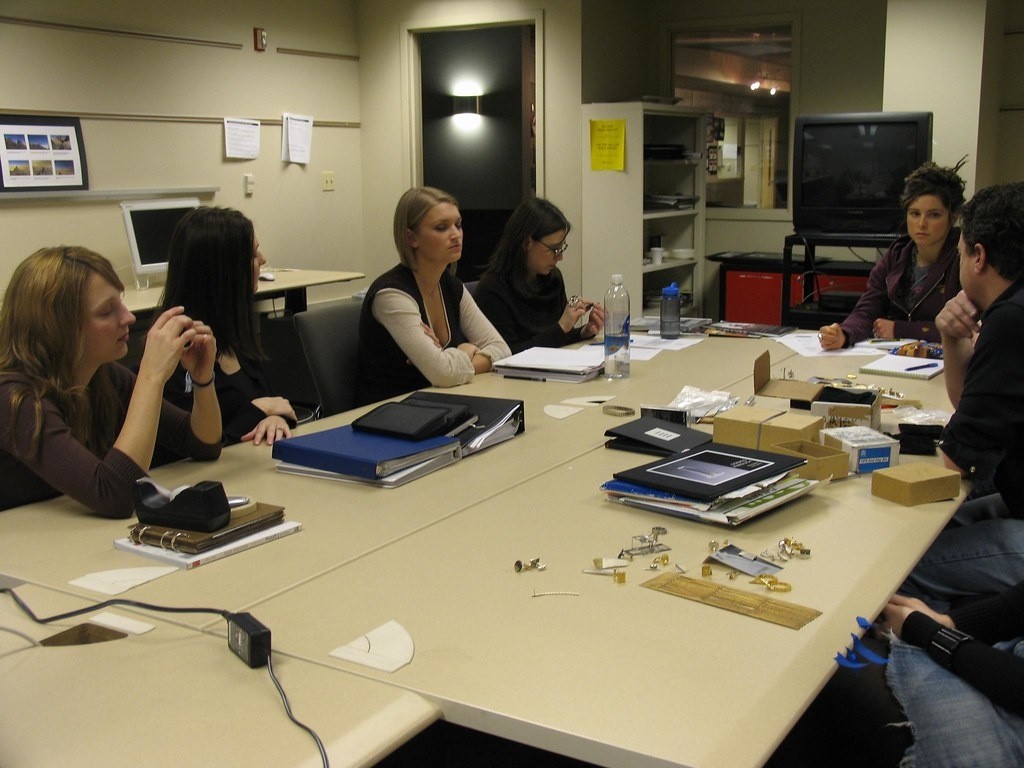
[659,11,803,220]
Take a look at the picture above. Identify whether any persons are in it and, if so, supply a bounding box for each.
[818,159,964,353]
[128,202,322,447]
[472,195,608,355]
[355,183,513,405]
[0,243,227,519]
[891,182,1024,653]
[874,595,1024,768]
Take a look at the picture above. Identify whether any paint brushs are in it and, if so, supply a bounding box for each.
[708,334,762,338]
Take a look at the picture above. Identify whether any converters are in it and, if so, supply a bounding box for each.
[227,612,271,668]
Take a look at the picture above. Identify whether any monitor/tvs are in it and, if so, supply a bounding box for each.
[119,197,201,289]
[792,111,933,242]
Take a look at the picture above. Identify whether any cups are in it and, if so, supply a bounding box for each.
[650,248,663,265]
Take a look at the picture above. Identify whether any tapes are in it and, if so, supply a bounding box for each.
[170,486,191,501]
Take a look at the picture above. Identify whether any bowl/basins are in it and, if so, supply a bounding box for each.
[672,249,694,260]
[645,251,669,258]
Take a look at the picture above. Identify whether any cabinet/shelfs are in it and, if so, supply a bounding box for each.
[783,234,896,332]
[579,102,708,320]
[718,261,879,327]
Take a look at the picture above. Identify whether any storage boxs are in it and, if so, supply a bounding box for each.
[871,461,961,507]
[712,406,824,452]
[753,348,882,430]
[818,425,901,473]
[770,440,849,481]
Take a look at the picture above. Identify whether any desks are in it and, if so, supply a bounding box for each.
[0,328,974,768]
[120,267,365,318]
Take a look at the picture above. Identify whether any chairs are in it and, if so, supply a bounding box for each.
[294,301,363,421]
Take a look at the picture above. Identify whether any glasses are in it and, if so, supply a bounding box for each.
[537,239,568,257]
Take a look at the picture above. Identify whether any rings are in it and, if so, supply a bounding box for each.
[568,295,579,306]
[818,333,823,341]
[277,427,284,430]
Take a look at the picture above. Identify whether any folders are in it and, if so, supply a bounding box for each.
[272,424,465,489]
[399,390,527,458]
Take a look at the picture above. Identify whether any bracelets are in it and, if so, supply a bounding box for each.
[189,372,215,387]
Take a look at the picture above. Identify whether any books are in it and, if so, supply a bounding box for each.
[646,142,692,162]
[114,503,301,570]
[597,440,834,525]
[859,354,945,380]
[642,190,698,212]
[491,346,605,385]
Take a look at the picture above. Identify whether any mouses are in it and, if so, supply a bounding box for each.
[259,273,274,281]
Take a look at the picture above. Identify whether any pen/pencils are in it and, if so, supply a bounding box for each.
[870,339,905,343]
[590,339,633,345]
[504,374,546,382]
[905,363,938,371]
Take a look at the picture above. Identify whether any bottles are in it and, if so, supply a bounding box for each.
[659,282,681,340]
[603,274,631,380]
